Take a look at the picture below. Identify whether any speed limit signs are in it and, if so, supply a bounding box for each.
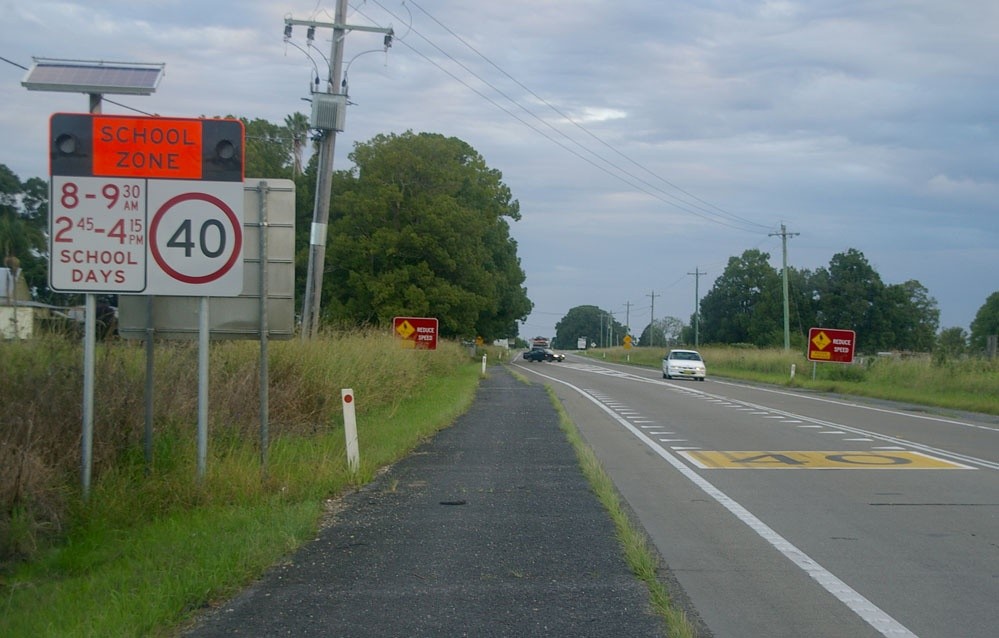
[150,191,241,284]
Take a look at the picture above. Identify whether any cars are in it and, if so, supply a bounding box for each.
[662,349,706,382]
[523,348,565,362]
